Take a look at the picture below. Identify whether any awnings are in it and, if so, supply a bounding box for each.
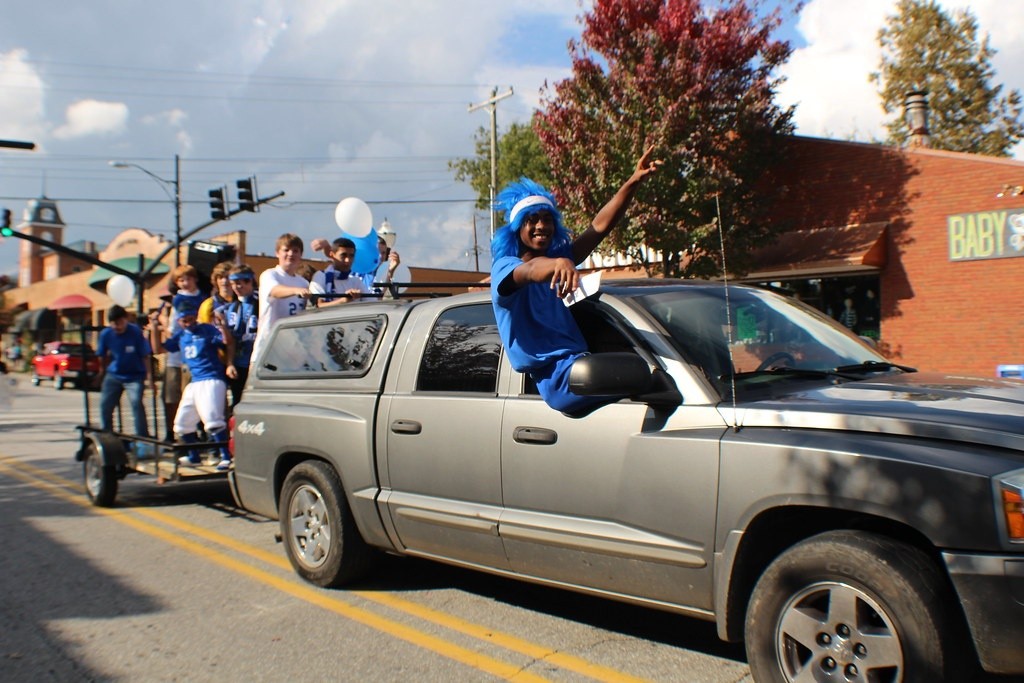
[89,256,170,288]
[51,293,92,308]
[7,306,57,332]
[679,220,888,284]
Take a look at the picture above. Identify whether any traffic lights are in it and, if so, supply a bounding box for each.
[0,209,12,237]
[237,177,255,212]
[209,188,225,220]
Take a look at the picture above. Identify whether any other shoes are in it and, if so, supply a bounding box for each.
[160,438,176,451]
[206,456,219,465]
[179,455,202,467]
[217,459,230,469]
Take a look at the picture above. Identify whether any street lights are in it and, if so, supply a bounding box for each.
[109,155,181,268]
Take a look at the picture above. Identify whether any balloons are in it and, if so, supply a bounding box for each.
[334,196,373,238]
[106,274,134,308]
[375,260,411,294]
[143,235,400,469]
[338,226,382,273]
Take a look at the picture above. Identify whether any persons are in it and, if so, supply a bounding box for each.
[96,305,151,441]
[490,144,658,410]
[866,290,877,321]
[841,299,858,331]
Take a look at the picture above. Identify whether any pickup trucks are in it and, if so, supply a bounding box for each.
[227,278,1024,683]
[31,342,100,390]
